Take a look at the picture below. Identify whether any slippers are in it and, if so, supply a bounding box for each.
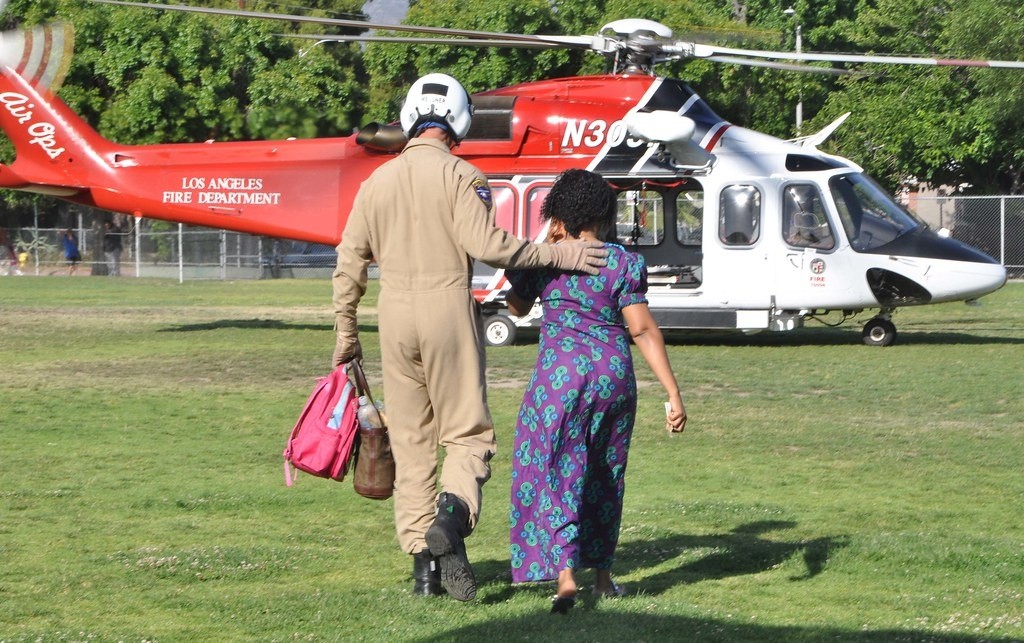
[590,581,629,598]
[549,594,575,615]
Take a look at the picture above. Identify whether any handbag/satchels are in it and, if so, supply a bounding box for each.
[349,356,396,500]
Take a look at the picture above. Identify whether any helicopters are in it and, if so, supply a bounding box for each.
[0,0,1024,346]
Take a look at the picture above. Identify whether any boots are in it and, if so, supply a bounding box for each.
[412,548,443,597]
[425,491,476,602]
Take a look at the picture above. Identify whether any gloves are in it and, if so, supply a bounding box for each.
[548,237,609,276]
[332,327,364,373]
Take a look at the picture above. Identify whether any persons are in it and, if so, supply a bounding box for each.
[103,219,123,277]
[936,215,954,238]
[64,228,82,276]
[331,72,609,603]
[504,167,688,611]
[268,237,282,279]
[1,246,28,276]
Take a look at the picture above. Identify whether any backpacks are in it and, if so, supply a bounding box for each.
[282,362,360,487]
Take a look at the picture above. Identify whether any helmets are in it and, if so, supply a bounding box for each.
[400,72,475,146]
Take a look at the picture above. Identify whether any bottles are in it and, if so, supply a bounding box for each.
[357,396,382,429]
